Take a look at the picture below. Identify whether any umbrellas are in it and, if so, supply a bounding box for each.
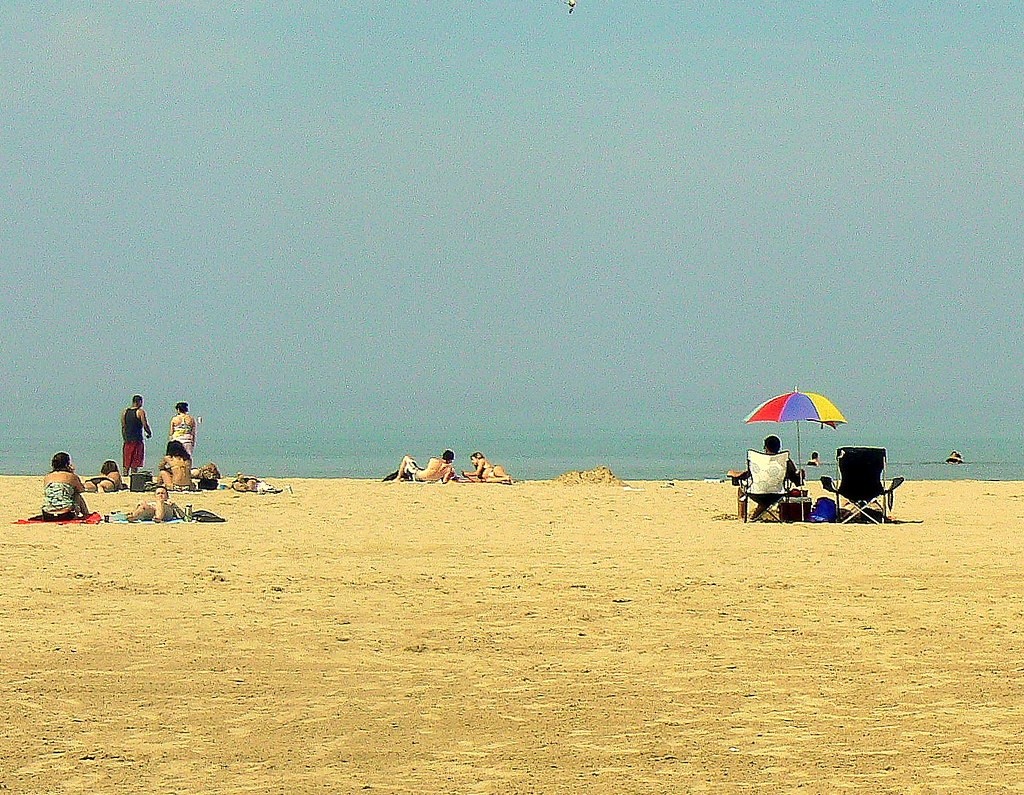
[741,385,848,469]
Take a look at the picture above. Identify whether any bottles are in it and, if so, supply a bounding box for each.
[185,504,192,517]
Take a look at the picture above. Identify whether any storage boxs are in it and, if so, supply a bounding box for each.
[779,501,813,523]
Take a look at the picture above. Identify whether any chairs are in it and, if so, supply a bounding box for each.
[727,447,794,526]
[819,445,905,525]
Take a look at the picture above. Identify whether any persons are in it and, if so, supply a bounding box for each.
[810,451,822,466]
[84,459,122,493]
[949,451,957,457]
[739,434,805,519]
[393,448,456,483]
[127,486,183,524]
[461,450,513,484]
[41,452,91,521]
[121,394,153,476]
[170,401,197,458]
[157,439,194,490]
[233,474,258,491]
